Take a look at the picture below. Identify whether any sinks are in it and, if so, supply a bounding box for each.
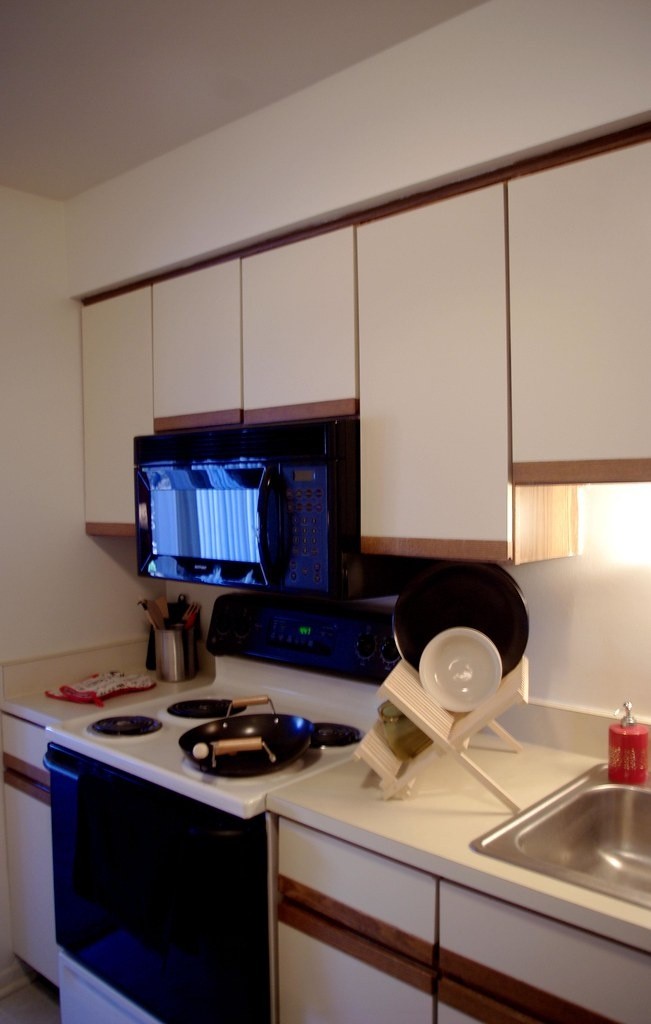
[467,782,650,910]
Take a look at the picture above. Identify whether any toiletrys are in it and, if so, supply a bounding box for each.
[607,702,648,786]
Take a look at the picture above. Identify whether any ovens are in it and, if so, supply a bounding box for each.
[42,740,276,1024]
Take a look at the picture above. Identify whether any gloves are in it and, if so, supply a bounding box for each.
[45,670,157,707]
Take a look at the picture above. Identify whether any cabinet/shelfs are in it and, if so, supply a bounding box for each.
[76,128,651,566]
[0,711,54,989]
[275,819,651,1024]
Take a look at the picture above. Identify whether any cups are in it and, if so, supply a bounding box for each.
[154,628,199,682]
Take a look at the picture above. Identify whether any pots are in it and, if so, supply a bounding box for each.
[178,694,316,779]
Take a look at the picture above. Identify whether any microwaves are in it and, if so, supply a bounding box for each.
[133,414,360,592]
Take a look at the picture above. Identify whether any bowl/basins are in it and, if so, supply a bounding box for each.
[417,627,503,714]
[391,561,530,677]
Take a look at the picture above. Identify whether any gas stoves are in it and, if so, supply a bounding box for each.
[44,588,408,819]
